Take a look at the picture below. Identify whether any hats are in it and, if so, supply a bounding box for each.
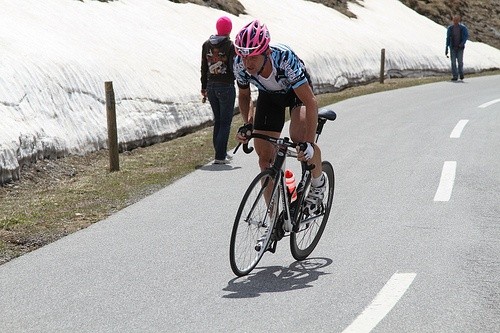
[216,16,233,35]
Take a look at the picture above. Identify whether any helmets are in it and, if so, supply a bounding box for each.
[234,20,270,57]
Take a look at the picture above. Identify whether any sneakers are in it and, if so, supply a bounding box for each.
[214,158,230,164]
[254,225,273,251]
[305,170,327,204]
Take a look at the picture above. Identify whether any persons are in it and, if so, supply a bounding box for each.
[200,16,238,165]
[232,19,329,251]
[445,15,468,82]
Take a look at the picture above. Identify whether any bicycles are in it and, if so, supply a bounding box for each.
[229,108,336,276]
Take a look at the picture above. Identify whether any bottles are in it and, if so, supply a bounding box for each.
[284,170,297,202]
